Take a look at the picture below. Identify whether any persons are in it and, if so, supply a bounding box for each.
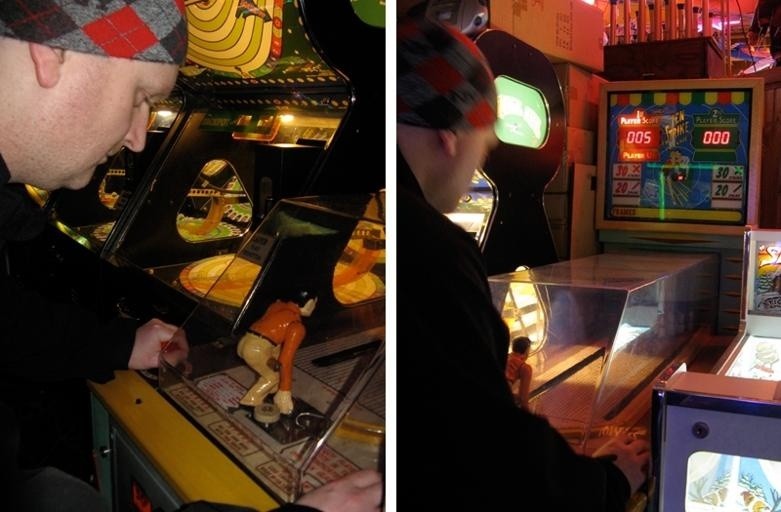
[396,17,650,512]
[236,288,319,416]
[0,0,385,512]
[505,336,532,412]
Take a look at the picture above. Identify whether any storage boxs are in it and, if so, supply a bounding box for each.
[487,0,608,262]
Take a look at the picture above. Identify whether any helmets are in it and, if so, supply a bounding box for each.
[397,10,500,133]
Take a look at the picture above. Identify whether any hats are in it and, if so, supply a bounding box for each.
[0,0,191,67]
